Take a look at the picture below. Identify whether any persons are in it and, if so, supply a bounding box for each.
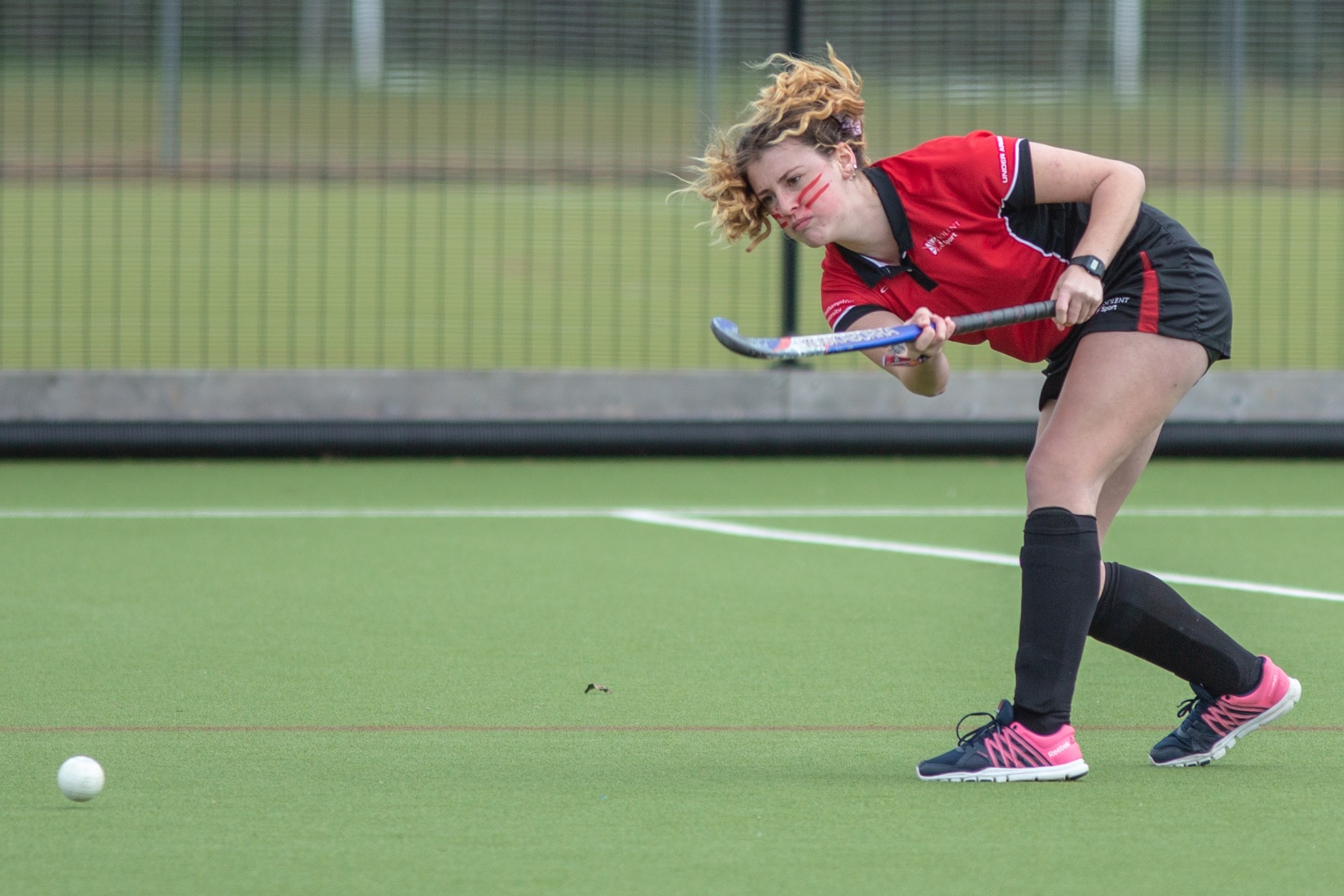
[669,37,1301,783]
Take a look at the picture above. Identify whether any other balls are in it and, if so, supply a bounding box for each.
[58,757,106,802]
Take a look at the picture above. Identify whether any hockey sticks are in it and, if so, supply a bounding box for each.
[712,299,1057,362]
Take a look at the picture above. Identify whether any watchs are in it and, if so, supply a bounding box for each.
[1067,254,1105,280]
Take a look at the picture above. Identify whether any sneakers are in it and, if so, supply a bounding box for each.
[1149,654,1302,769]
[916,699,1089,783]
